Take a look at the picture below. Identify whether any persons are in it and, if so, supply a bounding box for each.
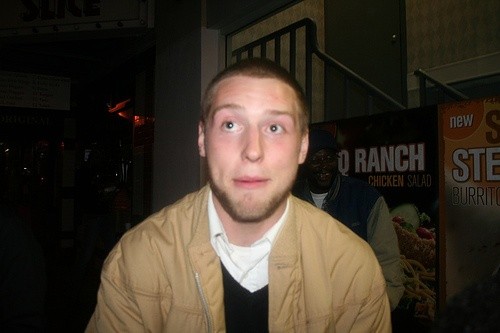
[291,129,403,313]
[85,57,392,333]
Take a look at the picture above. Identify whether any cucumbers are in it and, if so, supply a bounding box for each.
[390,203,423,233]
